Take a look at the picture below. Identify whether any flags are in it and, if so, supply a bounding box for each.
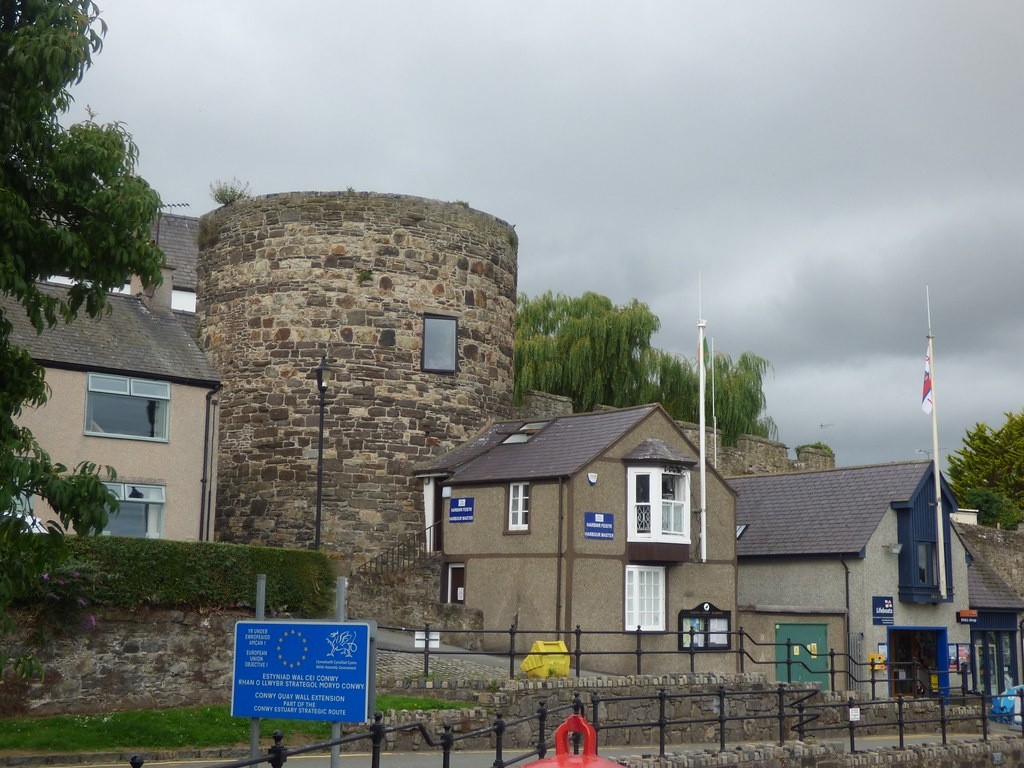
[921,345,932,414]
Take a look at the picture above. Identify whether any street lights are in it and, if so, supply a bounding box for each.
[310,353,338,552]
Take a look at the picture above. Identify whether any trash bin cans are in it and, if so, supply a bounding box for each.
[521,640,571,677]
